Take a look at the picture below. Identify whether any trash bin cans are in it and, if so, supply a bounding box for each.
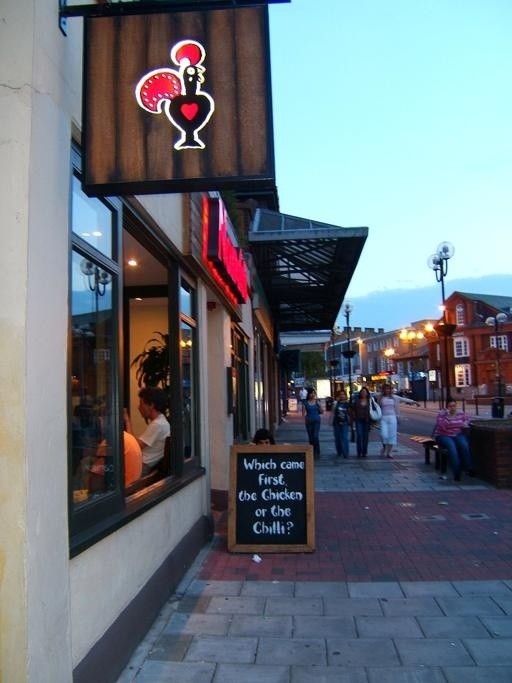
[491,397,504,418]
[326,397,334,411]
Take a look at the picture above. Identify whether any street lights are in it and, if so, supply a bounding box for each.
[385,348,395,395]
[486,312,510,399]
[328,322,340,398]
[343,301,356,404]
[80,258,112,324]
[400,329,425,399]
[181,340,192,375]
[428,239,454,407]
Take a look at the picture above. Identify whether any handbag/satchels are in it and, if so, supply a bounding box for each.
[370,402,382,421]
[349,409,355,420]
[319,406,323,414]
[328,416,335,425]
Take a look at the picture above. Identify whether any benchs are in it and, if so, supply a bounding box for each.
[411,424,458,471]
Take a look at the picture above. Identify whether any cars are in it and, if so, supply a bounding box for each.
[351,391,422,408]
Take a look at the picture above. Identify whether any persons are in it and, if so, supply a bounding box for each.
[375,382,422,457]
[328,388,353,457]
[477,381,488,395]
[124,385,173,477]
[302,387,324,458]
[352,386,378,457]
[249,428,292,445]
[299,386,309,403]
[435,397,474,482]
[288,388,296,398]
[81,404,146,496]
[469,384,478,398]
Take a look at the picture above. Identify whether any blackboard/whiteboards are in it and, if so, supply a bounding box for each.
[227,444,316,553]
